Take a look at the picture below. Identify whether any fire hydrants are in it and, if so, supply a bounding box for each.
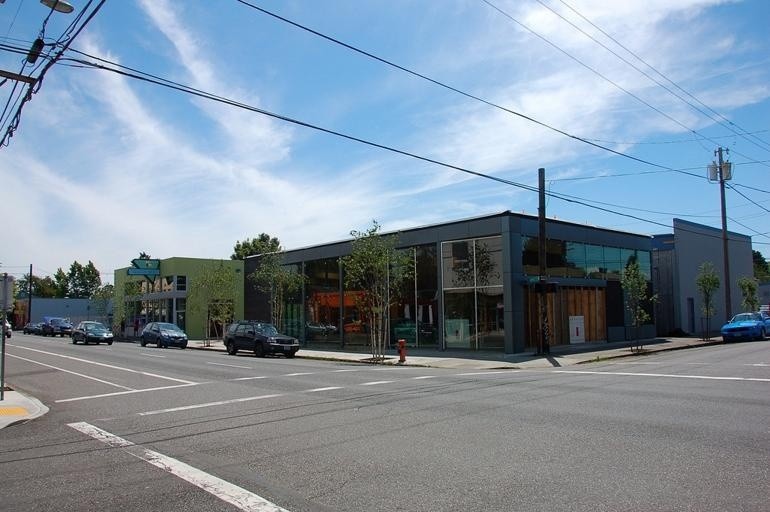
[397,339,407,362]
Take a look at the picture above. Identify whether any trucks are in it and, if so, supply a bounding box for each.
[0,312,12,338]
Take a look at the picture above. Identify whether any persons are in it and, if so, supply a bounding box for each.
[133,319,140,338]
[121,317,127,338]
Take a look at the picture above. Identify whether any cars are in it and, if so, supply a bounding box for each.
[720,310,770,344]
[140,322,188,349]
[305,320,337,336]
[24,317,73,337]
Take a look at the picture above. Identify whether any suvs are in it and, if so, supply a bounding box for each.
[71,321,114,345]
[223,320,300,358]
